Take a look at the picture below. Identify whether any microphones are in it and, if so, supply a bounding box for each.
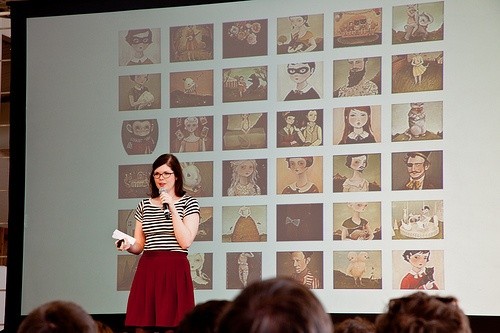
[159,187,170,220]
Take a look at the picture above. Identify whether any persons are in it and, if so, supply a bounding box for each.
[114,153,200,333]
[178,277,334,333]
[334,291,473,333]
[17,300,112,333]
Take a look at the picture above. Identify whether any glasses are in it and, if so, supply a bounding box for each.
[153,172,174,179]
